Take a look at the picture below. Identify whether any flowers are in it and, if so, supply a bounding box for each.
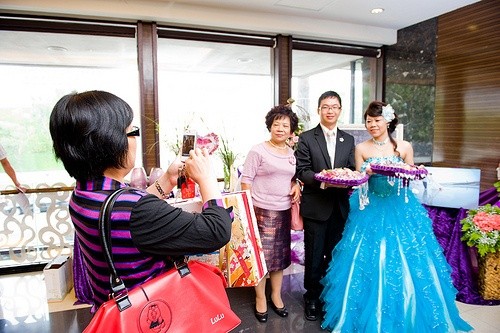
[460,203,500,257]
[286,122,303,150]
[381,104,395,122]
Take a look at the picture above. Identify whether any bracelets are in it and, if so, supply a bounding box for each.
[155,180,167,199]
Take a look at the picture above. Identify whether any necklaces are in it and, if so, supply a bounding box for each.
[372,137,390,146]
[269,139,286,150]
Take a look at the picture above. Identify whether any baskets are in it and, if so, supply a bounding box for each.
[478,251,500,301]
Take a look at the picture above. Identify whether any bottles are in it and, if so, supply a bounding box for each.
[149,168,164,187]
[132,168,146,189]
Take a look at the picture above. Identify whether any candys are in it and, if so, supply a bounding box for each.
[372,160,419,170]
[321,167,363,180]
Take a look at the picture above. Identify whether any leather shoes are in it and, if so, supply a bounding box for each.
[269,293,288,317]
[254,297,268,322]
[304,299,320,321]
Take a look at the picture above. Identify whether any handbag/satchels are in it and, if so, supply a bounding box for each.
[82,188,242,333]
[290,180,304,231]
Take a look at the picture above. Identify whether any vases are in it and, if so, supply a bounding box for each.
[478,251,500,300]
[223,164,236,193]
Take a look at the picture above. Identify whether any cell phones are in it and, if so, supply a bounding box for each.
[179,131,196,172]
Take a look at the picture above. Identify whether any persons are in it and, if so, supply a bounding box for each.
[350,101,416,333]
[241,105,300,322]
[0,143,28,193]
[49,92,235,316]
[291,91,356,320]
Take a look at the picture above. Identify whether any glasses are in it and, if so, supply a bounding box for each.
[319,105,341,111]
[127,125,140,137]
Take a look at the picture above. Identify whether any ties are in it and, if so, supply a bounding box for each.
[326,130,335,171]
